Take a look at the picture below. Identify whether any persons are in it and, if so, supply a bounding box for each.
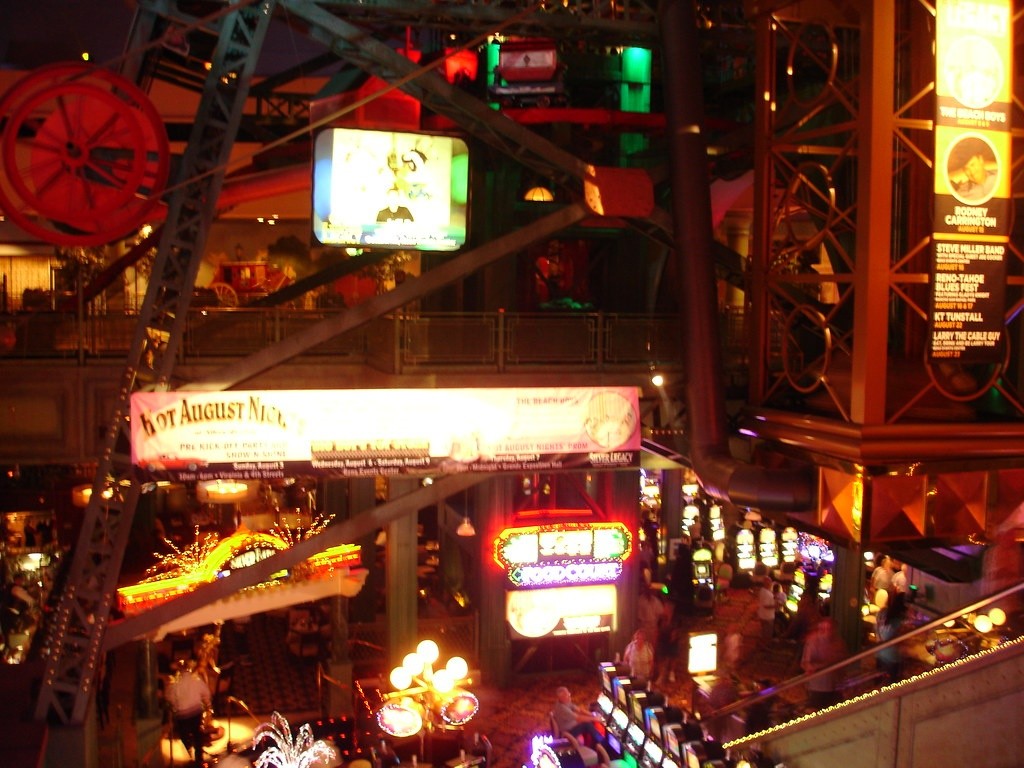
[743,679,772,734]
[689,516,701,538]
[874,592,909,685]
[955,139,998,201]
[0,574,31,654]
[795,561,827,622]
[801,615,850,710]
[376,189,414,223]
[639,591,680,686]
[622,631,653,683]
[642,511,658,556]
[718,556,732,579]
[760,576,792,642]
[870,553,907,603]
[234,244,248,261]
[171,660,211,768]
[556,687,607,746]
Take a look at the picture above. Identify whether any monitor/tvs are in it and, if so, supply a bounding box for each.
[312,125,472,254]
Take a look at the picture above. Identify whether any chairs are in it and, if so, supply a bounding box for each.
[548,711,613,768]
[369,728,494,768]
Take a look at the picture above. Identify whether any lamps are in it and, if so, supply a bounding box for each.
[456,487,475,536]
